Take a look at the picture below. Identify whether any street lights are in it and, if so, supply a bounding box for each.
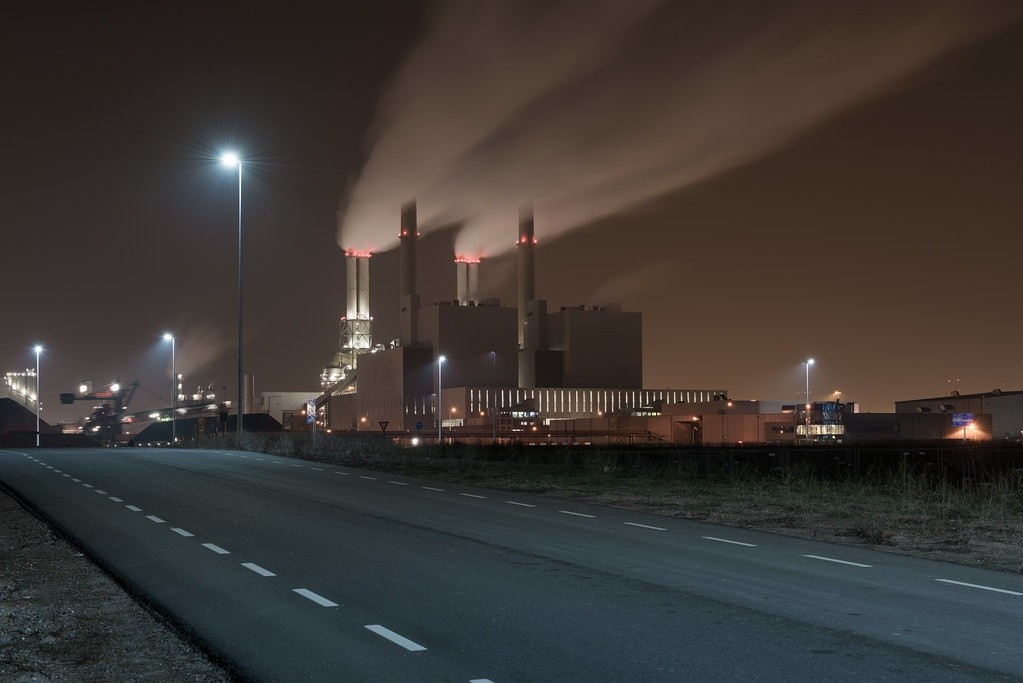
[33,344,43,449]
[162,333,176,448]
[438,355,446,446]
[802,359,815,440]
[221,152,245,448]
